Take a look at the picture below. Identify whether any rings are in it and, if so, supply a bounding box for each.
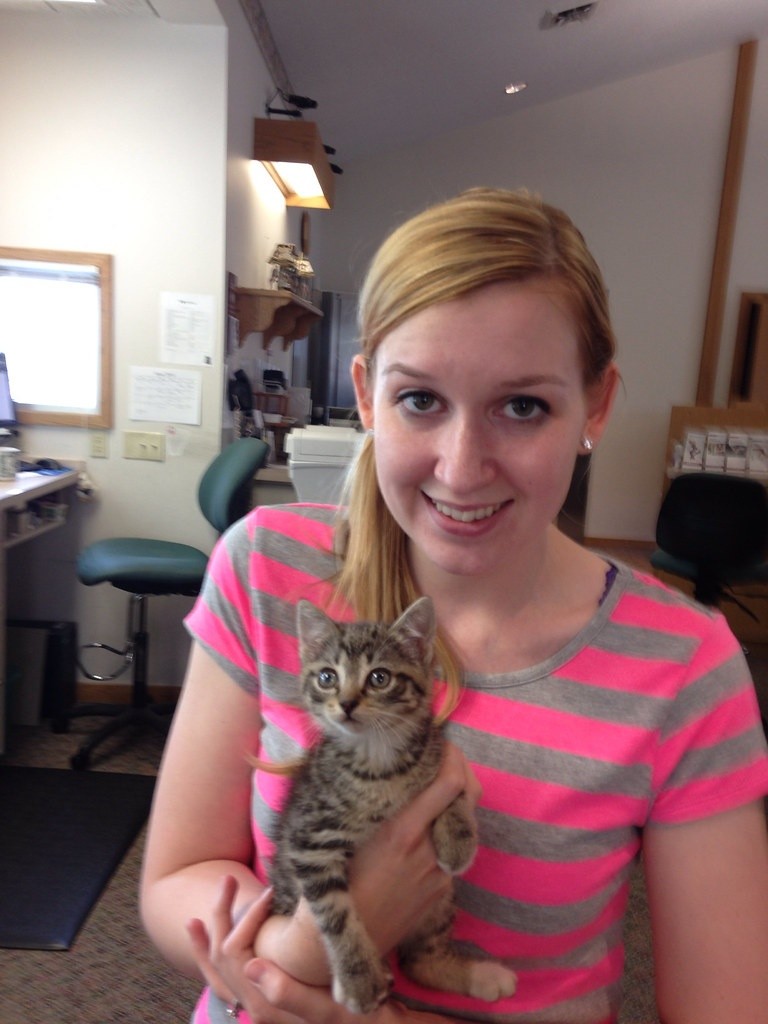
[226,1001,242,1019]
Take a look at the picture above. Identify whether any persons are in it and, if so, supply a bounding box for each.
[139,187,767,1024]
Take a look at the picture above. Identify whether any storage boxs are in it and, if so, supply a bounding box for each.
[680,426,768,476]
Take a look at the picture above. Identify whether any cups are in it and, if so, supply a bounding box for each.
[0,447,21,480]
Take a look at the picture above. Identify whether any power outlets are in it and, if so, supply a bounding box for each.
[88,430,109,458]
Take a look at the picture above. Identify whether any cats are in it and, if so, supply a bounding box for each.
[262,592,518,1017]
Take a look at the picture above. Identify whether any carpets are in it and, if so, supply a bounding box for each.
[0,767,156,951]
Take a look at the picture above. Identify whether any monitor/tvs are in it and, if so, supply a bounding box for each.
[0,353,17,427]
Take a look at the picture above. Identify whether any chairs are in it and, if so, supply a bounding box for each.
[50,437,270,771]
[650,473,768,629]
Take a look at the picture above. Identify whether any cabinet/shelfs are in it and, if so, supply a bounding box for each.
[235,287,325,430]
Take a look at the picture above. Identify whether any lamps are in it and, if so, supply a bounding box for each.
[254,118,333,210]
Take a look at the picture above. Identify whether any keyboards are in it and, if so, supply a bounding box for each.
[15,460,41,472]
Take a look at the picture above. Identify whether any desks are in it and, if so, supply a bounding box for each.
[250,467,299,509]
[0,457,85,551]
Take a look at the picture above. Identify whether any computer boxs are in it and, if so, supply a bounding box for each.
[5,619,78,726]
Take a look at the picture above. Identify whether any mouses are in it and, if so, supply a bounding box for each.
[36,458,61,470]
